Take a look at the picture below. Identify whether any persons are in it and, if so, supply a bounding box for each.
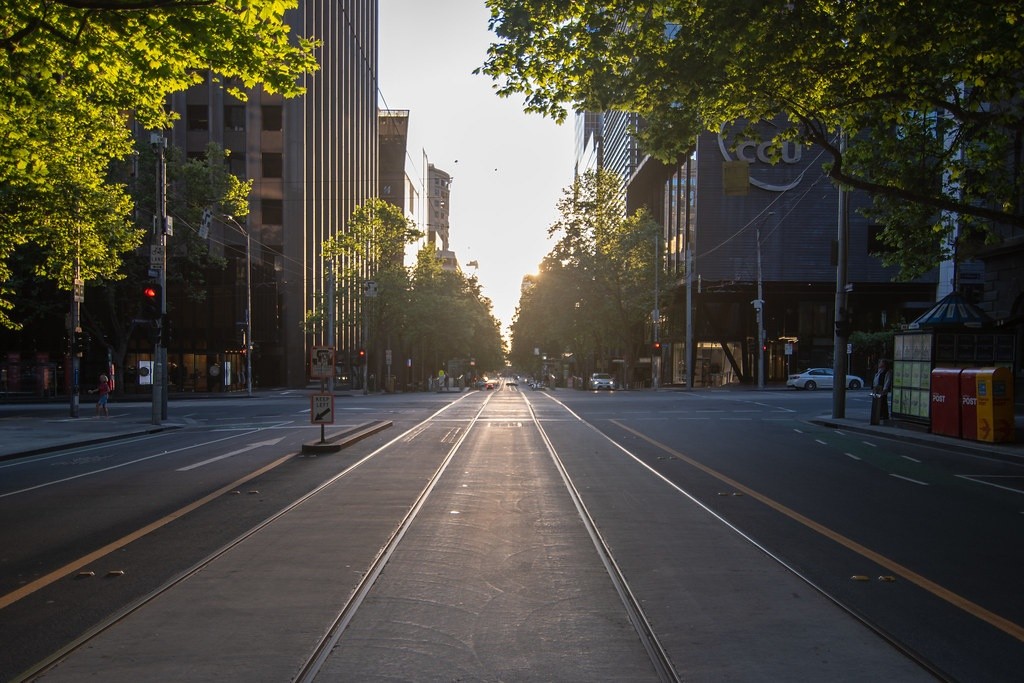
[443,371,450,391]
[88,375,110,419]
[871,357,891,419]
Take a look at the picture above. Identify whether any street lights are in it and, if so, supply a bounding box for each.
[755,211,776,390]
[225,214,252,394]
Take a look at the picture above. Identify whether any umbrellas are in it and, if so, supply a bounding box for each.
[909,291,994,368]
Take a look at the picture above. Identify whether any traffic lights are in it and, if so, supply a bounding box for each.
[653,342,661,358]
[763,343,768,354]
[142,284,162,318]
[358,350,366,367]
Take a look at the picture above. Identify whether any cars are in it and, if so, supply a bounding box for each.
[787,368,864,390]
[589,373,616,390]
[487,384,494,389]
